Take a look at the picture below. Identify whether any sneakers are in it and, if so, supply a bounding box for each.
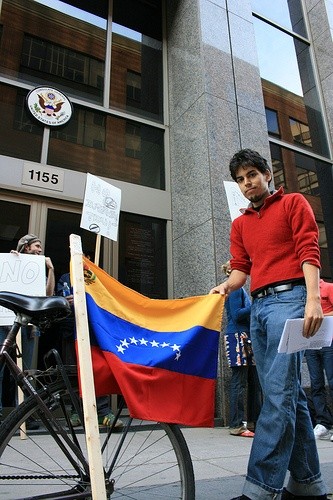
[98,411,123,429]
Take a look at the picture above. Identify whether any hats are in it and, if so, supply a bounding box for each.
[16,233,41,253]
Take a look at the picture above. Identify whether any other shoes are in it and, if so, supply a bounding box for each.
[65,413,82,427]
[25,418,40,429]
[312,424,331,440]
[281,487,327,500]
[231,494,251,500]
[240,430,255,437]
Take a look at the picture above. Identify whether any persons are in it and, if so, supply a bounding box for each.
[0,234,55,429]
[57,251,123,426]
[208,148,327,500]
[221,259,260,437]
[304,277,333,441]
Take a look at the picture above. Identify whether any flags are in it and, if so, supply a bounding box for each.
[70,256,225,427]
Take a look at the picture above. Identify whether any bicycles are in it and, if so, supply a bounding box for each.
[0,292,198,499]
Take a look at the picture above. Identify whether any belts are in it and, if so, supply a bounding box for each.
[251,281,306,298]
